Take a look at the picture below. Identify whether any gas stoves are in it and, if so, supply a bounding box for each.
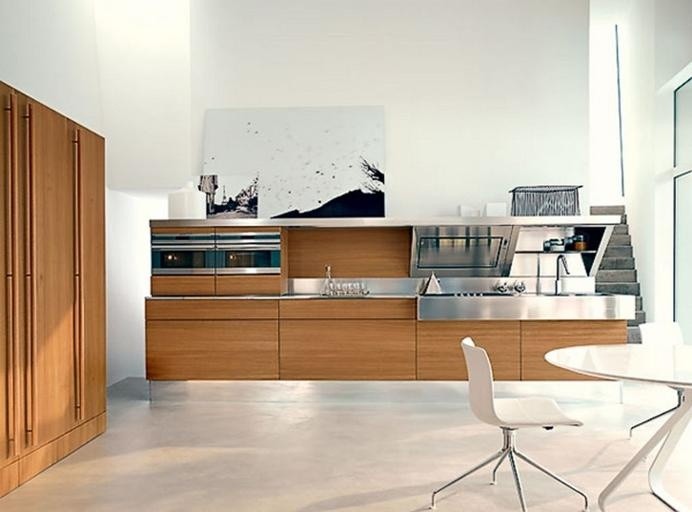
[416,292,515,296]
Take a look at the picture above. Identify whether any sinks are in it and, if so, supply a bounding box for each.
[545,292,614,297]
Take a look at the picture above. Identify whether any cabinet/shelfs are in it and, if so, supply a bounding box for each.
[152,224,293,300]
[281,299,419,380]
[0,81,104,500]
[417,322,520,379]
[522,323,627,377]
[145,298,278,380]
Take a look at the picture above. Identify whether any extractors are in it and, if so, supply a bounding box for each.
[408,226,516,278]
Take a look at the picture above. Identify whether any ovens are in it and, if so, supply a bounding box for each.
[151,233,281,274]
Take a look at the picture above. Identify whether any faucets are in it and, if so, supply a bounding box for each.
[555,255,570,295]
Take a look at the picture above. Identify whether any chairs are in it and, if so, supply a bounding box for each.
[427,330,593,512]
[625,318,690,440]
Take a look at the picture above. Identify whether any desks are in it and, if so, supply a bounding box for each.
[539,343,691,512]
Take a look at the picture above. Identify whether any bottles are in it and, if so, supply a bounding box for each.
[542,234,586,253]
[319,265,369,296]
